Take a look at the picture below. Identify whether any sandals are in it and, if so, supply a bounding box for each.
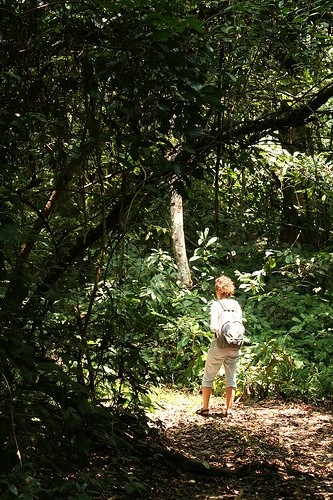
[196,408,210,417]
[226,412,232,418]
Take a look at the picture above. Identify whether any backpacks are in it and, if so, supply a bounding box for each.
[217,299,245,351]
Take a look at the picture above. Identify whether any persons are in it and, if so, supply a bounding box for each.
[196,275,242,417]
